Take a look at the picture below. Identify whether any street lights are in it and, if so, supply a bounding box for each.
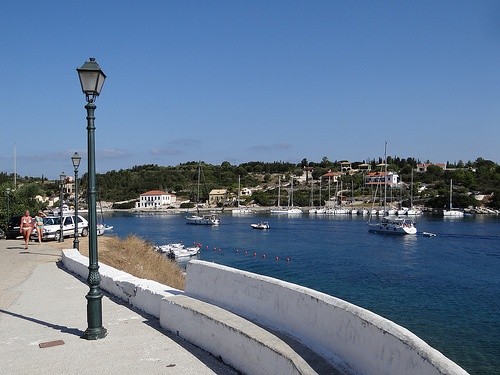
[290,176,293,209]
[71,152,82,251]
[76,58,106,340]
[58,171,66,242]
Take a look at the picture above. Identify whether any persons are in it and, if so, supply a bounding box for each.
[20,209,46,249]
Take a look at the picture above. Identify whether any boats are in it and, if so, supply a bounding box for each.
[422,231,437,237]
[474,206,500,216]
[270,210,288,213]
[443,210,464,216]
[288,209,303,214]
[102,223,114,231]
[251,221,270,229]
[153,243,200,258]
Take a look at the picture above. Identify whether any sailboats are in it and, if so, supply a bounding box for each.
[184,160,222,225]
[308,168,422,215]
[366,141,418,235]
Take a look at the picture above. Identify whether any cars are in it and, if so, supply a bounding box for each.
[6,205,88,241]
